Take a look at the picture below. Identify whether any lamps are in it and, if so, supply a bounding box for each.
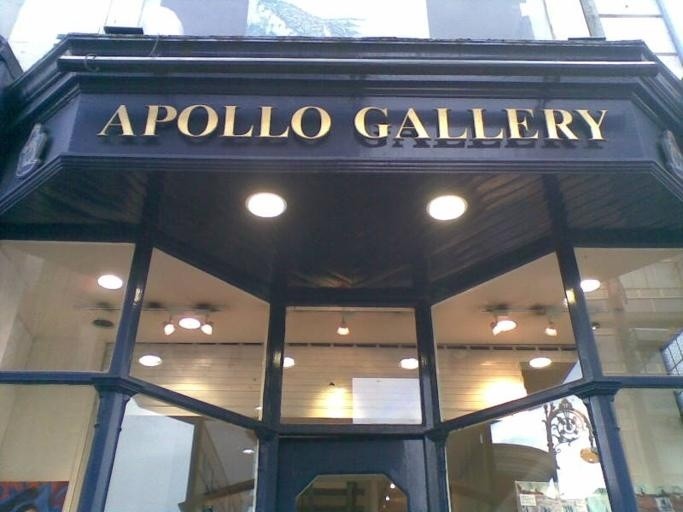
[489,316,518,336]
[178,314,202,331]
[336,314,350,336]
[545,317,558,335]
[201,313,214,336]
[162,315,177,338]
[592,321,600,332]
